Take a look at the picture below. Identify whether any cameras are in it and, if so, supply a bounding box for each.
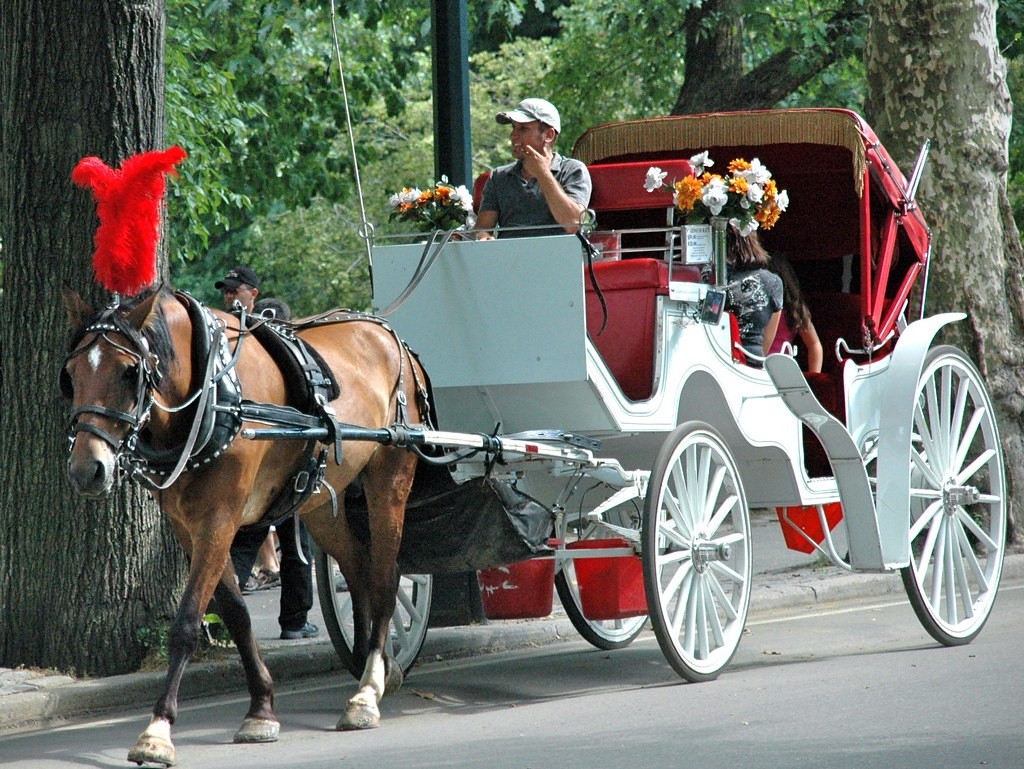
[701,290,726,325]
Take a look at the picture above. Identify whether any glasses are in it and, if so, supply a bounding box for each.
[222,287,255,295]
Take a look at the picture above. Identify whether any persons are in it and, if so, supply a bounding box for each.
[470,96,594,243]
[201,296,319,645]
[701,212,824,378]
[213,266,282,593]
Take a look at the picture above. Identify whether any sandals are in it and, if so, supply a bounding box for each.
[256,569,283,590]
[243,573,259,590]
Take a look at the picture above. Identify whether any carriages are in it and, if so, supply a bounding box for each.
[56,94,1010,767]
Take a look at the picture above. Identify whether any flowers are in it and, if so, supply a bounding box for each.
[642,150,789,236]
[388,175,479,242]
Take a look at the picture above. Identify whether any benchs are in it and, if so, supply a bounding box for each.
[474,160,785,403]
[793,291,894,454]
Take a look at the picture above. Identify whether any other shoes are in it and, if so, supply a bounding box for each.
[333,571,348,593]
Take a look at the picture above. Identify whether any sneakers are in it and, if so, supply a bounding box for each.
[282,621,319,639]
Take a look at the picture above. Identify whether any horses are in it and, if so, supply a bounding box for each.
[61,277,427,769]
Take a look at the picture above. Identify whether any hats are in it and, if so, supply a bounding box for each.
[495,97,561,133]
[214,267,257,288]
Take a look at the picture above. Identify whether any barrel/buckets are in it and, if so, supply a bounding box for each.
[568,481,650,619]
[477,537,563,618]
